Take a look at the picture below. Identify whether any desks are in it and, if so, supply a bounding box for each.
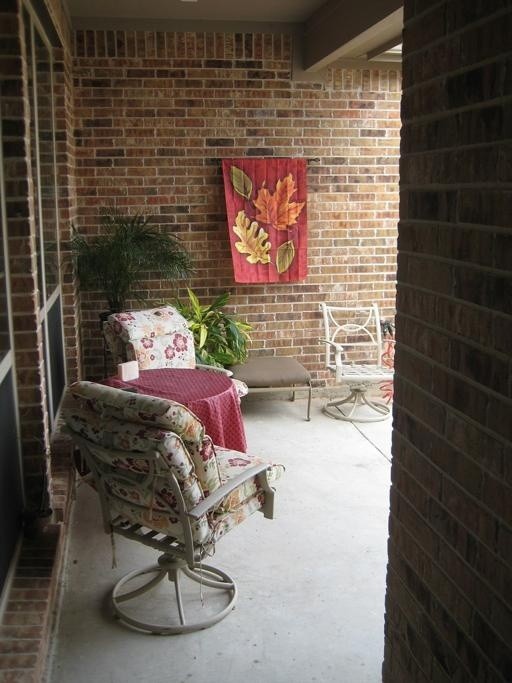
[102,364,248,452]
[228,351,313,420]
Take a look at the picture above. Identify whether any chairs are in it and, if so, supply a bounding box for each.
[313,298,393,420]
[101,305,246,406]
[58,379,285,632]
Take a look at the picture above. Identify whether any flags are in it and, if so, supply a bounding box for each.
[221,156,308,284]
